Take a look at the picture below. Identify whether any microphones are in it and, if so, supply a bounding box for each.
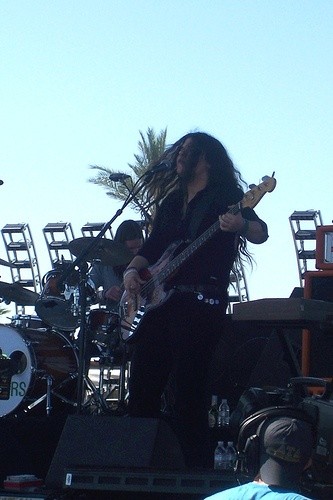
[109,173,130,182]
[145,160,172,175]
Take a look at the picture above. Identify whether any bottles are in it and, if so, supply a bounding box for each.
[207,395,220,430]
[219,399,230,427]
[225,441,237,472]
[214,441,226,471]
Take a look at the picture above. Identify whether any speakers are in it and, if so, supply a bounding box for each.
[302,271,333,393]
[43,415,188,490]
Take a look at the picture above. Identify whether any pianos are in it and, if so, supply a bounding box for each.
[227,296,332,417]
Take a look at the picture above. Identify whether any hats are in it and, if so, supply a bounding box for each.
[259,416,315,488]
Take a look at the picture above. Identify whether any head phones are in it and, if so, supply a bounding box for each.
[237,405,317,467]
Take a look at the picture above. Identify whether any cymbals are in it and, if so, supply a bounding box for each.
[68,236,128,265]
[0,258,18,271]
[0,280,41,307]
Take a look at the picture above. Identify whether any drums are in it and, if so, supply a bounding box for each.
[0,322,88,420]
[81,308,121,360]
[35,265,99,332]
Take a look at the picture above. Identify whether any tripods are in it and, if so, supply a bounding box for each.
[14,318,113,418]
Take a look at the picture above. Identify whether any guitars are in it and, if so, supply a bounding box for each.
[118,173,276,343]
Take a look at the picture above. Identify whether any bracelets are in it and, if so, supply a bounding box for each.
[243,220,249,233]
[123,267,137,277]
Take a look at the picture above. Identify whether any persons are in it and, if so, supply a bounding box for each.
[90,220,144,311]
[123,132,269,468]
[203,417,314,500]
